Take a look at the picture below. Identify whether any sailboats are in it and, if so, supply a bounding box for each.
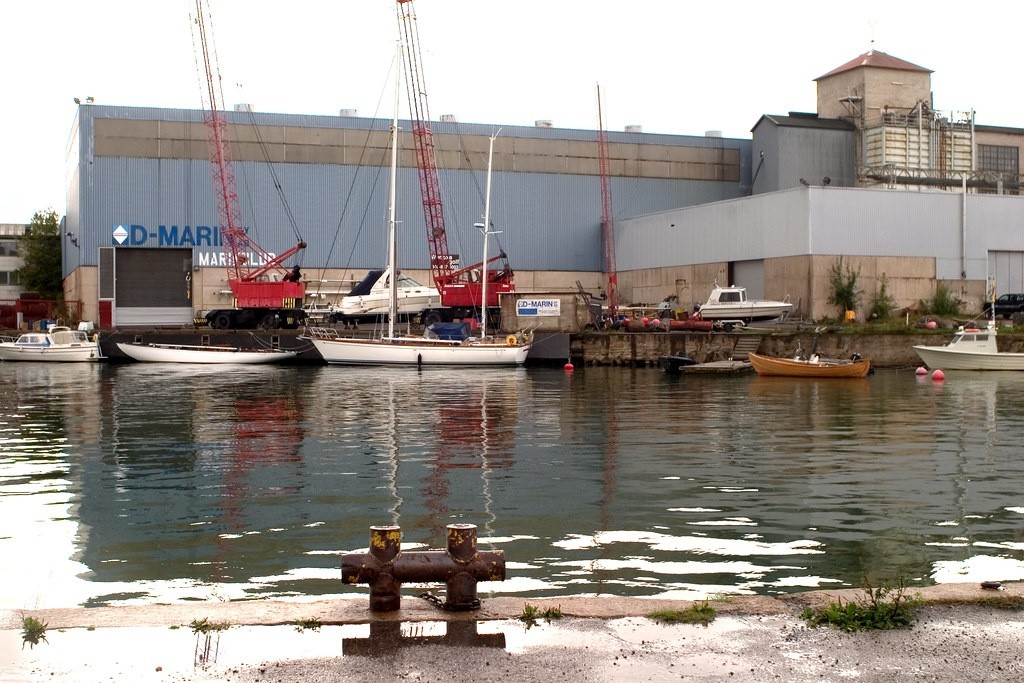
[298,40,534,366]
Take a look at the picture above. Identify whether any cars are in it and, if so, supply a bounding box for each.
[982,292,1024,321]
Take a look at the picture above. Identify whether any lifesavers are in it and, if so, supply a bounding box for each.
[506,335,516,345]
[965,329,980,333]
[93,334,97,341]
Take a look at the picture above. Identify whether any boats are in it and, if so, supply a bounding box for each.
[913,275,1024,372]
[116,333,299,364]
[697,284,792,323]
[747,337,871,379]
[0,324,102,362]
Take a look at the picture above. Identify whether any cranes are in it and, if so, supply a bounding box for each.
[395,0,518,307]
[594,81,620,325]
[185,0,308,312]
